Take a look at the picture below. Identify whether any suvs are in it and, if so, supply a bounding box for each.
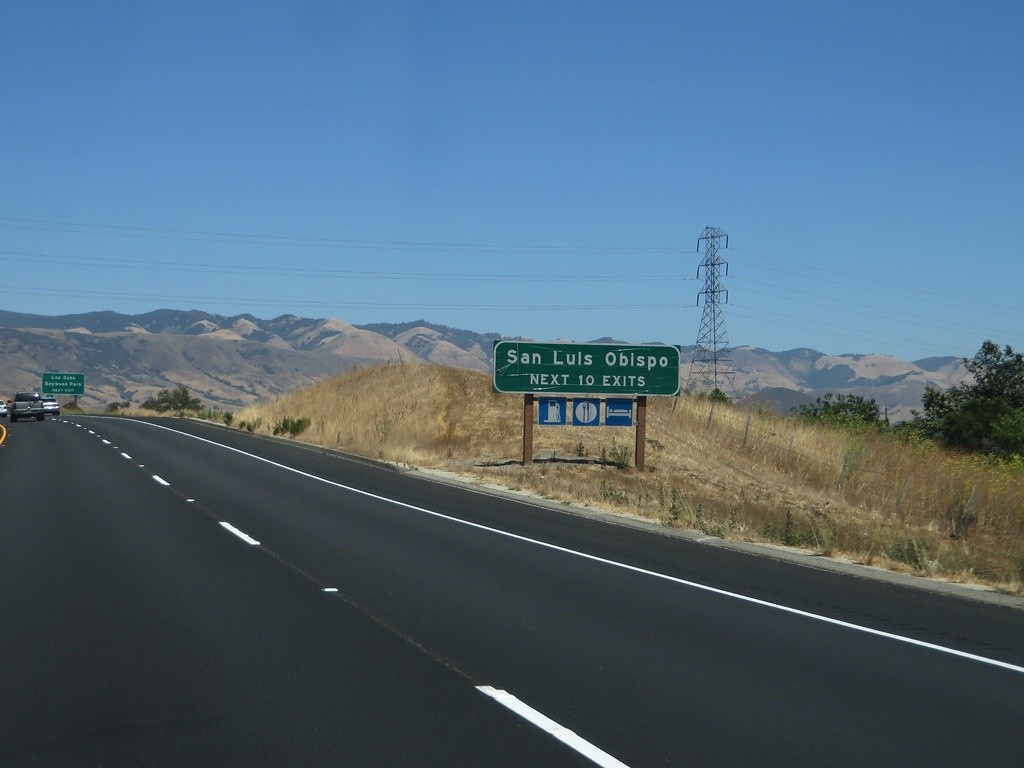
[11,392,44,423]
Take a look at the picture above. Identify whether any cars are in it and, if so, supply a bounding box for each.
[42,398,60,416]
[0,400,13,417]
